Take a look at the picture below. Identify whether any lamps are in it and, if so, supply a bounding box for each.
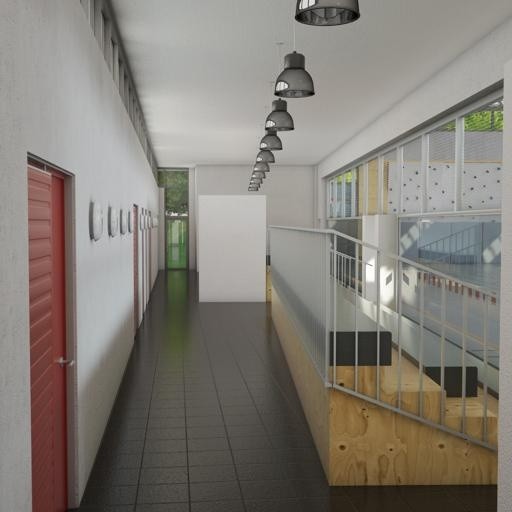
[248,0,360,192]
